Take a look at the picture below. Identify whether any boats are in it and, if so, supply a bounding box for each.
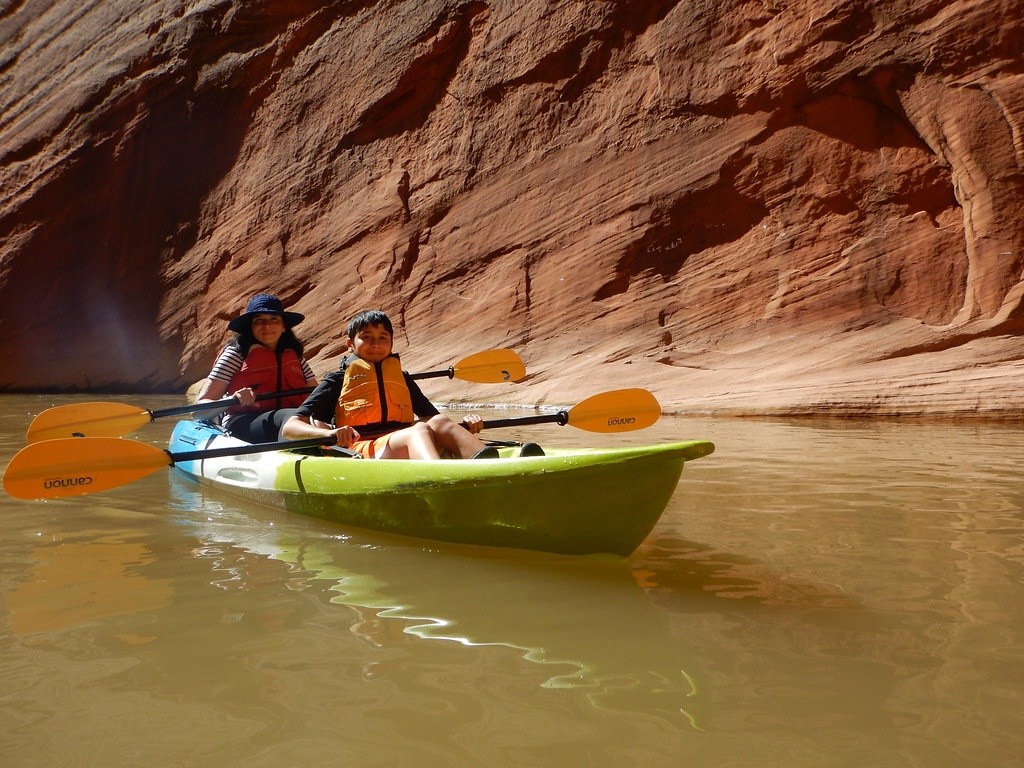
[168,418,715,555]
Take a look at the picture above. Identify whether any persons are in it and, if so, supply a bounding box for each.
[281,310,546,461]
[195,294,330,450]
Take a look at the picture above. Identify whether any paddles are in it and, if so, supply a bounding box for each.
[1,387,662,501]
[25,349,527,447]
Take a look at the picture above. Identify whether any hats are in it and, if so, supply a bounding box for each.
[228,294,305,334]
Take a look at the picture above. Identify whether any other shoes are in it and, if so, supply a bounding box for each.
[469,446,500,458]
[519,443,546,457]
